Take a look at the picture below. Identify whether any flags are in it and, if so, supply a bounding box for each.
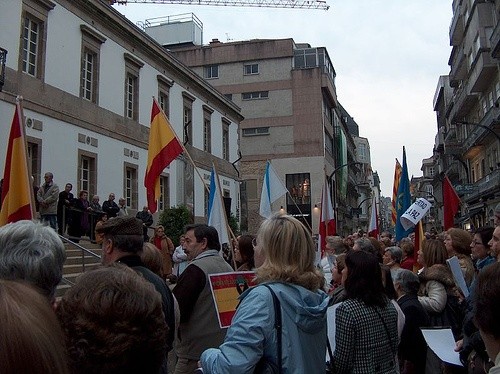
[368,201,379,239]
[258,162,288,218]
[145,100,184,214]
[318,182,337,257]
[391,150,424,272]
[0,105,33,227]
[443,177,460,231]
[207,168,229,244]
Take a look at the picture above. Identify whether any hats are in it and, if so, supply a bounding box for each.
[95,216,144,235]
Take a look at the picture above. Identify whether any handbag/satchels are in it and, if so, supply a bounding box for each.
[255,357,283,374]
[424,301,465,331]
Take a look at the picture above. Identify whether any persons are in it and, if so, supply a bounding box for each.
[117,197,128,218]
[37,172,59,230]
[136,206,154,241]
[149,225,174,281]
[67,191,92,243]
[0,213,500,374]
[88,195,102,244]
[172,234,190,278]
[102,192,120,220]
[57,183,74,236]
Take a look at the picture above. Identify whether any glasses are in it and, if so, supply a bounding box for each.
[252,238,258,248]
[474,240,483,245]
